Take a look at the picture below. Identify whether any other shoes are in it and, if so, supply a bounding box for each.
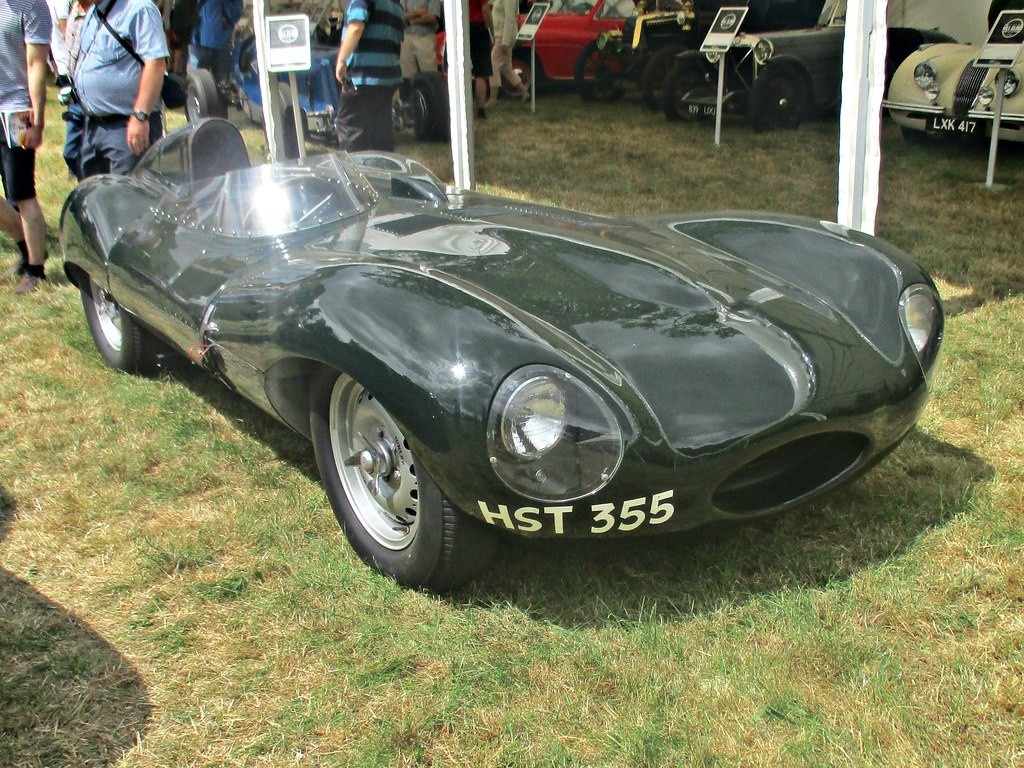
[485,101,498,107]
[478,107,487,120]
[12,274,42,294]
[520,93,529,103]
[0,248,49,281]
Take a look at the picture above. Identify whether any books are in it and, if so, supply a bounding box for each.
[0,109,34,149]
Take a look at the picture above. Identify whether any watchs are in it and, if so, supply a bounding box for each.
[132,110,150,122]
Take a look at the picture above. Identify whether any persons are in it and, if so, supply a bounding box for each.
[44,0,244,183]
[0,0,52,295]
[336,0,404,152]
[486,0,530,108]
[74,0,170,179]
[469,0,496,119]
[400,1,441,78]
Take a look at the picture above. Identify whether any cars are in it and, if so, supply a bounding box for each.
[61,115,943,595]
[883,10,1023,150]
[573,0,840,104]
[436,0,687,102]
[641,0,957,130]
[185,28,450,160]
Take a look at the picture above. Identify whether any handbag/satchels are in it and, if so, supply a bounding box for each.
[161,72,188,109]
[169,0,198,40]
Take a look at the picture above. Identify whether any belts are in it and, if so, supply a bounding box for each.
[89,110,161,126]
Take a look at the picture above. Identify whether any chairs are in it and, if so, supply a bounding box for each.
[187,120,252,221]
